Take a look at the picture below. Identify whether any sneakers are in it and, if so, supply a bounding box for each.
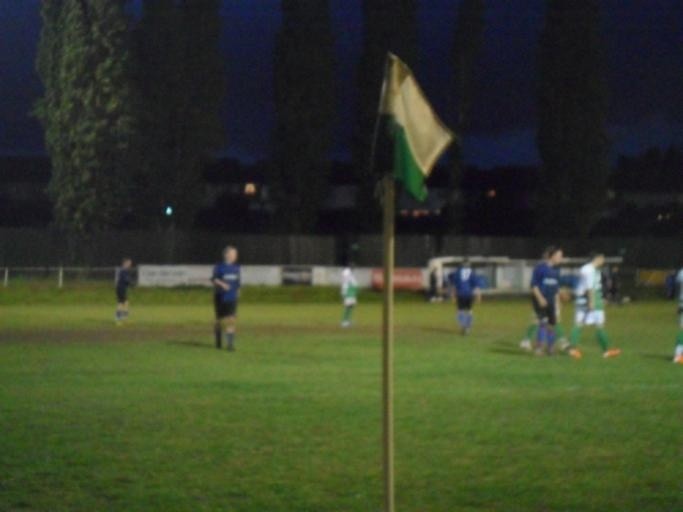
[673,354,683,364]
[601,347,621,359]
[518,337,583,358]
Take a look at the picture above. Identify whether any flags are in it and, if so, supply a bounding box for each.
[369,50,459,206]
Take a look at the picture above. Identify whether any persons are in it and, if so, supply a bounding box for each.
[558,250,622,360]
[527,246,564,358]
[428,266,444,302]
[518,248,569,351]
[112,253,136,323]
[209,243,245,354]
[672,268,683,365]
[338,260,358,328]
[450,256,484,337]
